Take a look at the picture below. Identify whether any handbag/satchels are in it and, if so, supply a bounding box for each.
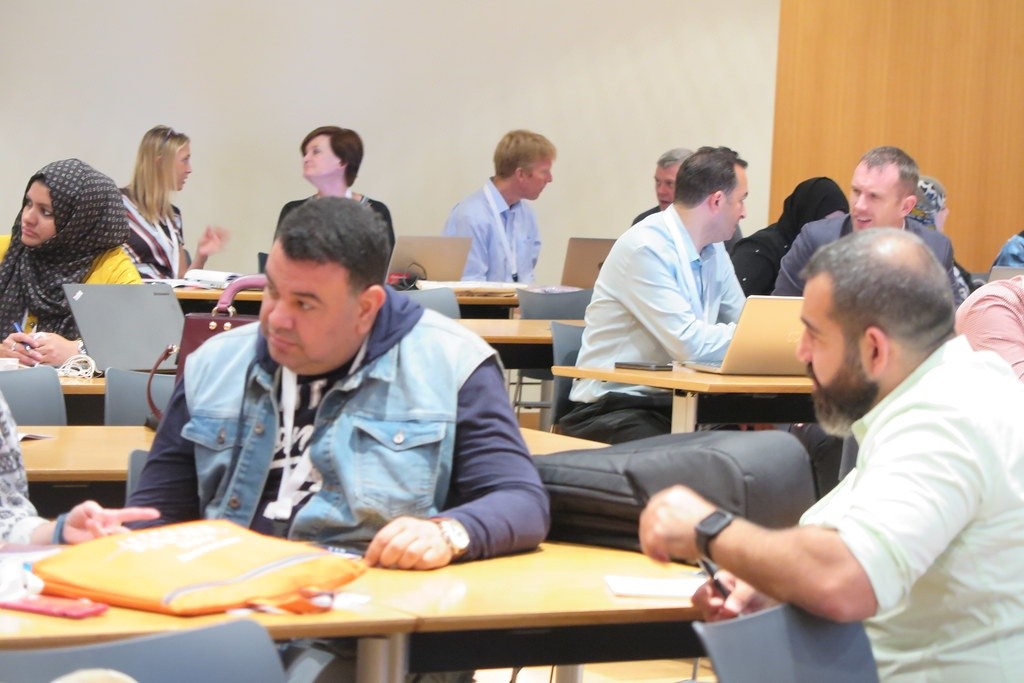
[33,517,370,615]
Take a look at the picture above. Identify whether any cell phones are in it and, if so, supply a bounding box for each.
[615,363,672,370]
[1,593,107,618]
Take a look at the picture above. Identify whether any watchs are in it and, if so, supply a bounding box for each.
[694,509,736,561]
[432,518,470,559]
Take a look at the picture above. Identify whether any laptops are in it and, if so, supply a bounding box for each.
[562,238,616,292]
[385,236,470,284]
[988,266,1024,284]
[62,283,186,373]
[685,296,806,378]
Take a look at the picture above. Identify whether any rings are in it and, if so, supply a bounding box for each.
[11,341,18,351]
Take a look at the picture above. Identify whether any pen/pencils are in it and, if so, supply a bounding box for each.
[13,322,30,351]
[701,559,729,600]
[185,278,213,283]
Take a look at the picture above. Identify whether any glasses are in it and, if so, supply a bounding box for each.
[160,127,173,153]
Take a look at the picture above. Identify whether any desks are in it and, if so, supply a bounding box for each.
[59,377,106,426]
[0,427,817,683]
[550,365,817,434]
[174,288,520,306]
[459,320,586,368]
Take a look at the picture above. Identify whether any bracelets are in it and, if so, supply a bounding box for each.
[53,513,65,544]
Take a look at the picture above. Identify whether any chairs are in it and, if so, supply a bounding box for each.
[691,602,881,683]
[0,619,290,683]
[457,286,593,434]
[0,365,174,506]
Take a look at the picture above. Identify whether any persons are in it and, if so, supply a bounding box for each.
[0,158,145,368]
[0,390,160,546]
[631,147,743,253]
[955,274,1024,379]
[121,196,550,683]
[118,127,228,284]
[989,229,1024,274]
[562,145,748,442]
[442,128,558,281]
[638,226,1024,683]
[272,126,396,285]
[770,145,970,420]
